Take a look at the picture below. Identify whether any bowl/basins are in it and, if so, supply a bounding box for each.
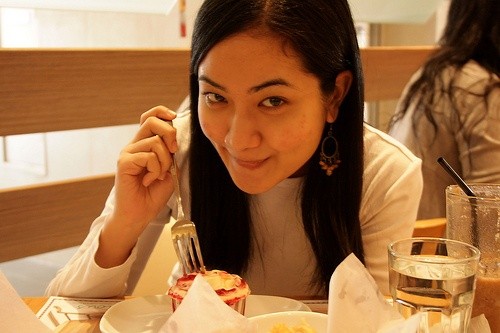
[248,311,329,333]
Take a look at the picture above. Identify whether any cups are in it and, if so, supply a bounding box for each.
[387,237,481,333]
[445,184,500,333]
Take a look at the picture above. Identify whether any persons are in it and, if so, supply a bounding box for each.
[387,0,500,221]
[45,0,423,299]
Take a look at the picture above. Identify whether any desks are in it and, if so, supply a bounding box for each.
[22,296,393,333]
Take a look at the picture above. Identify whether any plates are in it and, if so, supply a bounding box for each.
[99,294,313,333]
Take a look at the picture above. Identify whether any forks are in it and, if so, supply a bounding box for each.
[169,119,205,276]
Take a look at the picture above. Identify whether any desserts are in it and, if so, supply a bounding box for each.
[166,266,251,317]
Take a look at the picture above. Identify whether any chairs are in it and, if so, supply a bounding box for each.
[408,218,448,289]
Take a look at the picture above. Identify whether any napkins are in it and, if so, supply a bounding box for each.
[327,252,493,333]
[157,274,264,333]
[0,270,53,333]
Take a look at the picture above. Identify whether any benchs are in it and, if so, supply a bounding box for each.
[0,45,441,265]
